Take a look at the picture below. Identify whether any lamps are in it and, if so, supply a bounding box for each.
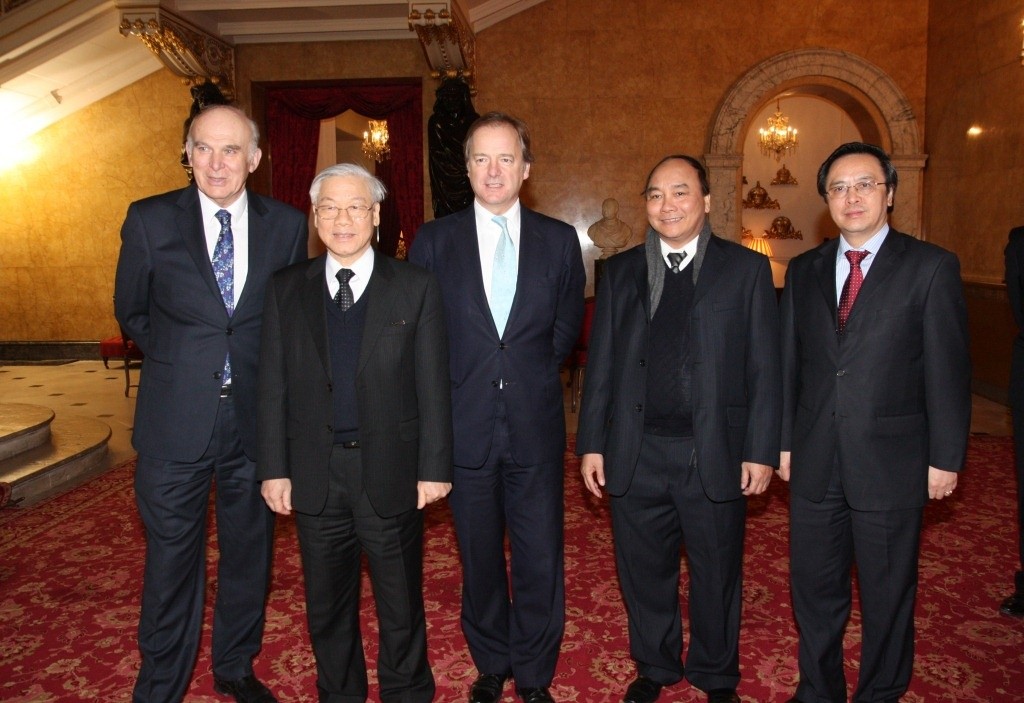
[746,239,774,259]
[361,119,391,163]
[757,97,800,162]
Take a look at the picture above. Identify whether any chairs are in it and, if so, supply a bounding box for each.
[114,296,142,397]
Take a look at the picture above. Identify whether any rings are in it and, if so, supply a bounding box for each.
[945,491,952,495]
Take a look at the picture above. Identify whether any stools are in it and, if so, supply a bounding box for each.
[100,336,143,368]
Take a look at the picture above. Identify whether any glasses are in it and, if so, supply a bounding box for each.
[826,179,887,199]
[314,200,379,220]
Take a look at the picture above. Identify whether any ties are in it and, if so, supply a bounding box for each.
[667,252,687,274]
[490,216,519,340]
[334,268,355,313]
[838,251,870,327]
[211,210,236,387]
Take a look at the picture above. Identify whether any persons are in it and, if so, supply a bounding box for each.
[778,163,791,182]
[574,156,782,702]
[428,76,483,219]
[406,109,587,703]
[1000,226,1024,618]
[255,163,451,703]
[114,105,309,703]
[780,143,971,703]
[180,80,230,184]
[587,197,632,248]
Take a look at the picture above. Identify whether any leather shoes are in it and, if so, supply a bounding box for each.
[999,590,1024,616]
[707,687,742,703]
[213,675,278,703]
[467,667,512,703]
[513,683,555,703]
[623,663,686,703]
